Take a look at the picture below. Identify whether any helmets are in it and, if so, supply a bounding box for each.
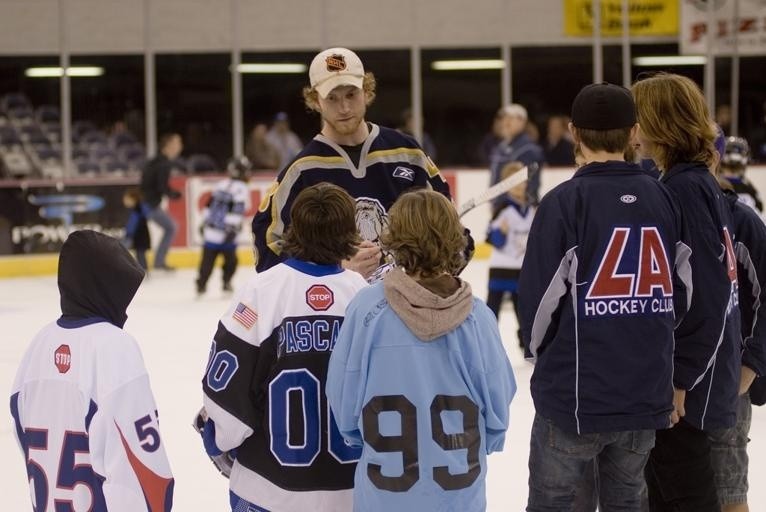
[723,136,750,168]
[228,157,253,180]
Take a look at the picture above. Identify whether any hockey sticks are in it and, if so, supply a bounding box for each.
[457,163,541,218]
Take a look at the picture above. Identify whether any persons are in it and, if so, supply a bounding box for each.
[122,110,303,294]
[323,190,516,511]
[194,182,372,511]
[8,230,175,512]
[480,74,766,511]
[252,48,451,279]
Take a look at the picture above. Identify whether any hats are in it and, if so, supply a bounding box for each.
[572,81,637,130]
[309,47,365,100]
